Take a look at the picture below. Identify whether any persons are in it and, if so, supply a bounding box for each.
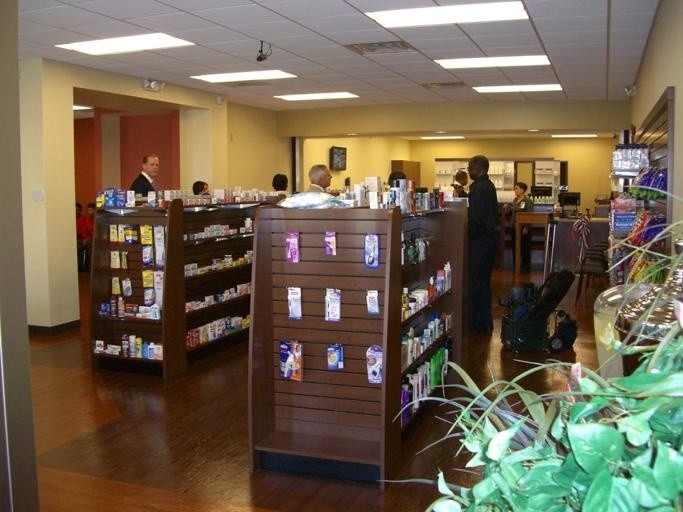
[76,202,84,271]
[192,181,209,195]
[451,171,469,197]
[82,202,97,272]
[129,153,160,197]
[386,170,406,189]
[306,164,333,193]
[450,154,501,336]
[501,181,534,274]
[272,173,290,196]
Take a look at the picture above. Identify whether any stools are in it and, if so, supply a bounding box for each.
[571,237,611,308]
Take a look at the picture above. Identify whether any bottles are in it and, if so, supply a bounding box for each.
[528,195,555,205]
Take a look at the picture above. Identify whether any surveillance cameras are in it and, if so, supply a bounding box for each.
[256,52,267,62]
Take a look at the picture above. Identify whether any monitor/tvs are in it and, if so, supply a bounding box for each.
[330,146,347,171]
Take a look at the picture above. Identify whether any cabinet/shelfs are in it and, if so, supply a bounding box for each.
[90,187,266,384]
[436,159,560,275]
[248,195,465,491]
